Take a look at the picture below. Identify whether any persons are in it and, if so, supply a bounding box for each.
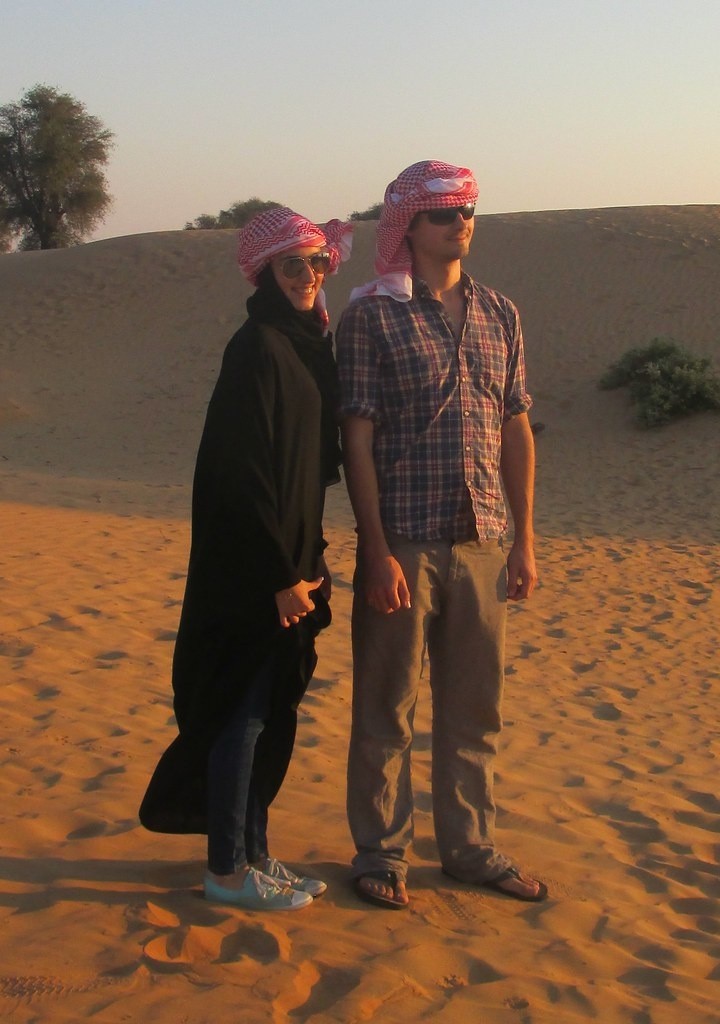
[139,204,356,911]
[334,159,550,911]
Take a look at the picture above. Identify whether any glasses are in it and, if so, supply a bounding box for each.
[417,203,476,226]
[271,252,331,279]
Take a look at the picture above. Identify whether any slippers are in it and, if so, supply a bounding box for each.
[352,853,409,910]
[441,862,548,901]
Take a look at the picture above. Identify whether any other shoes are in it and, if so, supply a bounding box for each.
[205,859,327,910]
